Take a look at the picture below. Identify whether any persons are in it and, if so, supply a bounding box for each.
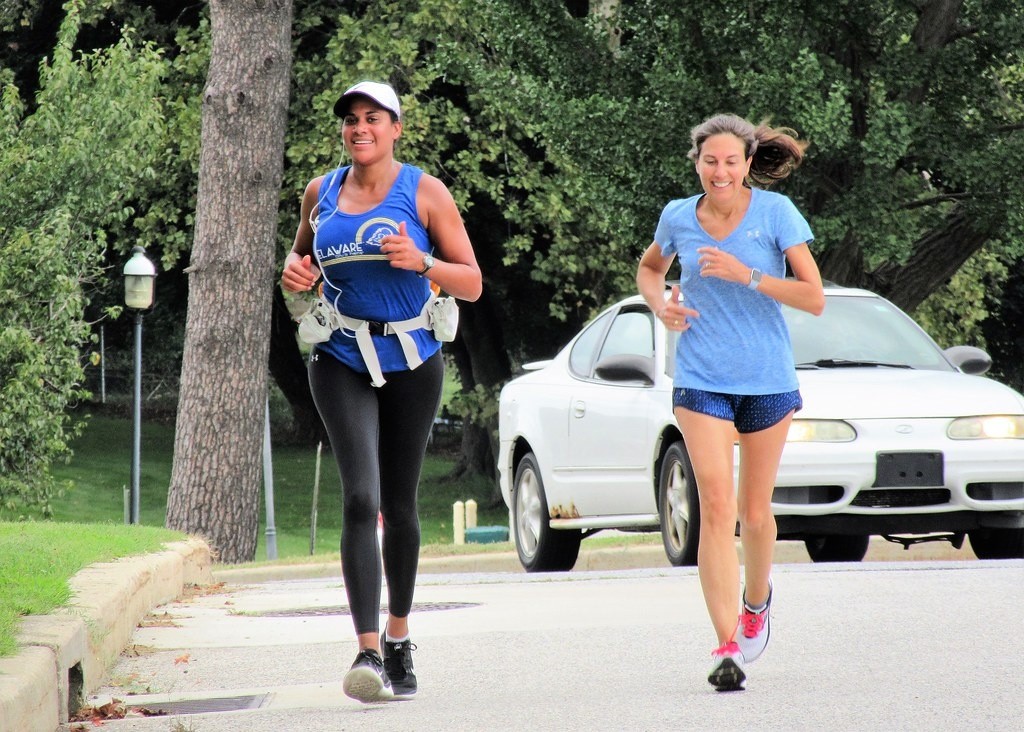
[282,81,482,705]
[637,111,825,691]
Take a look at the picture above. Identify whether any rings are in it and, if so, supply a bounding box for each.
[673,320,679,327]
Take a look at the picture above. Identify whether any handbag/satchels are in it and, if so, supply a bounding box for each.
[297,301,335,344]
[428,296,459,343]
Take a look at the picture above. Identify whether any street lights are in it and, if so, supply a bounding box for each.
[123,245,158,527]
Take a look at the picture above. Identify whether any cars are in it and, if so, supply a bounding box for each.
[497,279,1024,573]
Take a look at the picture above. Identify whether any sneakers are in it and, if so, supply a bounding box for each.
[708,619,746,692]
[379,621,417,700]
[342,648,394,704]
[739,577,773,662]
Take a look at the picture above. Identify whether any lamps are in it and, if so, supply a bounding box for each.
[124,248,156,309]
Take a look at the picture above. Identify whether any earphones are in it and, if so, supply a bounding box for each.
[315,215,320,225]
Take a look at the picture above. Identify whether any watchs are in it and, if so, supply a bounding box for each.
[747,268,762,290]
[416,252,435,275]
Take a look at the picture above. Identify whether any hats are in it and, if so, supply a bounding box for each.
[332,82,400,121]
